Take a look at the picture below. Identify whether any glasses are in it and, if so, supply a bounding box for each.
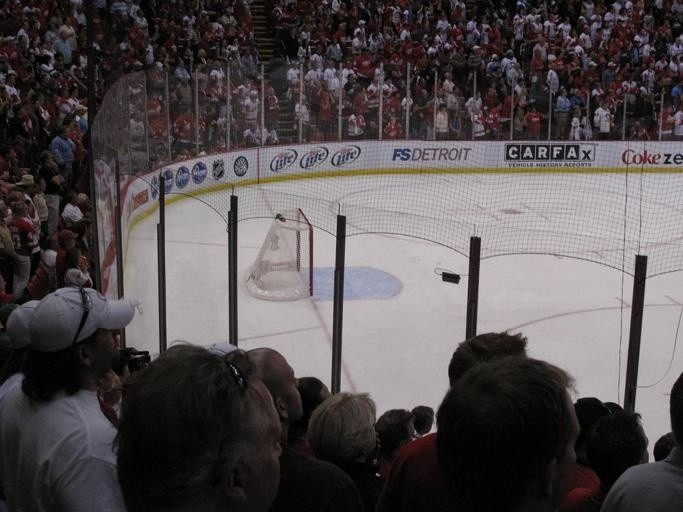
[70,286,91,350]
[209,354,249,409]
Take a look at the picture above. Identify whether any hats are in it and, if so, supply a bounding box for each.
[57,229,78,242]
[28,285,135,354]
[6,299,40,349]
[14,174,39,187]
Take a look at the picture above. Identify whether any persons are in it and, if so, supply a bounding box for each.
[1,2,682,510]
